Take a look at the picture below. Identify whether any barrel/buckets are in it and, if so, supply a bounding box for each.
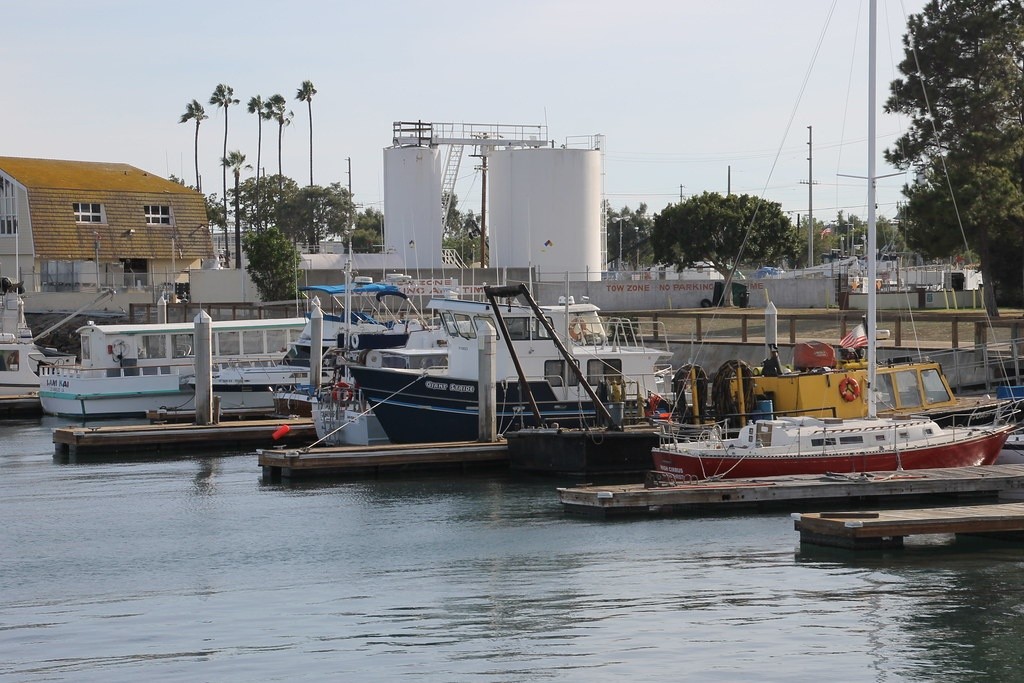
[604,402,625,426]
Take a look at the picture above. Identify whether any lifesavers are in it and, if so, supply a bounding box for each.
[568,317,586,340]
[839,377,860,402]
[112,339,130,357]
[351,333,360,349]
[332,382,352,406]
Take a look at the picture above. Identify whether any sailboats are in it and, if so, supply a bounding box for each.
[2,186,1024,484]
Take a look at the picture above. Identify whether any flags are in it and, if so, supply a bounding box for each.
[840,323,868,349]
[821,224,831,239]
